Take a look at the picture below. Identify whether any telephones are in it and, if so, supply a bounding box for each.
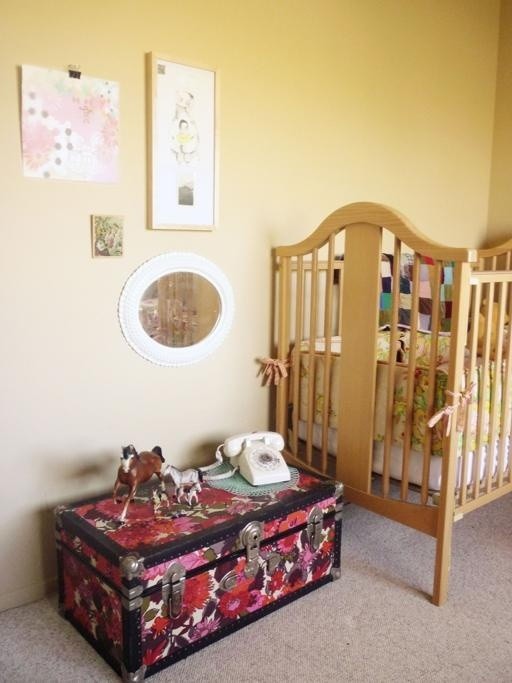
[223,431,291,487]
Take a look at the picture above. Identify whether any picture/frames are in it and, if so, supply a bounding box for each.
[143,50,218,232]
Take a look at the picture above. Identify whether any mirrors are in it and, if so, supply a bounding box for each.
[117,250,237,369]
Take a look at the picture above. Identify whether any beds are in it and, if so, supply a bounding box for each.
[274,201,512,608]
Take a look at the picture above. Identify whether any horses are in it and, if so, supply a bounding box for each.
[112,444,166,525]
[163,464,203,496]
[176,482,202,506]
